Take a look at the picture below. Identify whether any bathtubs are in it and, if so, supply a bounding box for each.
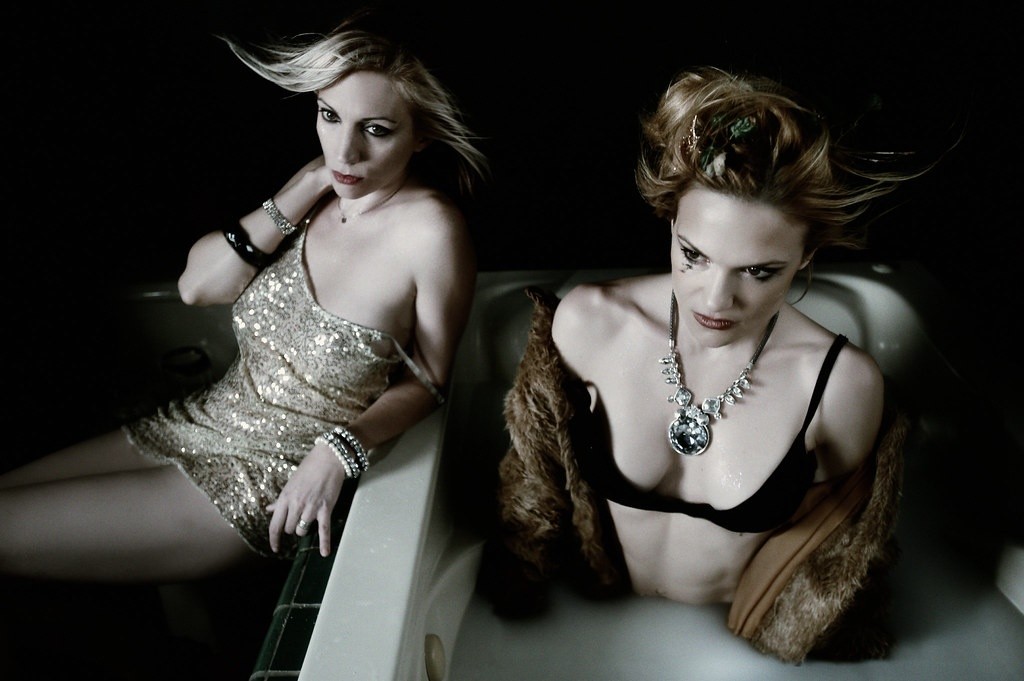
[298,259,1023,681]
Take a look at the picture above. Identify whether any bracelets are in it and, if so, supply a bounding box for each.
[314,426,370,479]
[222,219,277,269]
[262,197,298,234]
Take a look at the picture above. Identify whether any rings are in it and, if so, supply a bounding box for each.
[297,519,311,529]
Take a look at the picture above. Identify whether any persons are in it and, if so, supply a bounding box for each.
[490,66,912,665]
[0,0,482,590]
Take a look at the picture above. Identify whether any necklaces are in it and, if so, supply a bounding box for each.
[337,185,399,223]
[658,290,778,455]
